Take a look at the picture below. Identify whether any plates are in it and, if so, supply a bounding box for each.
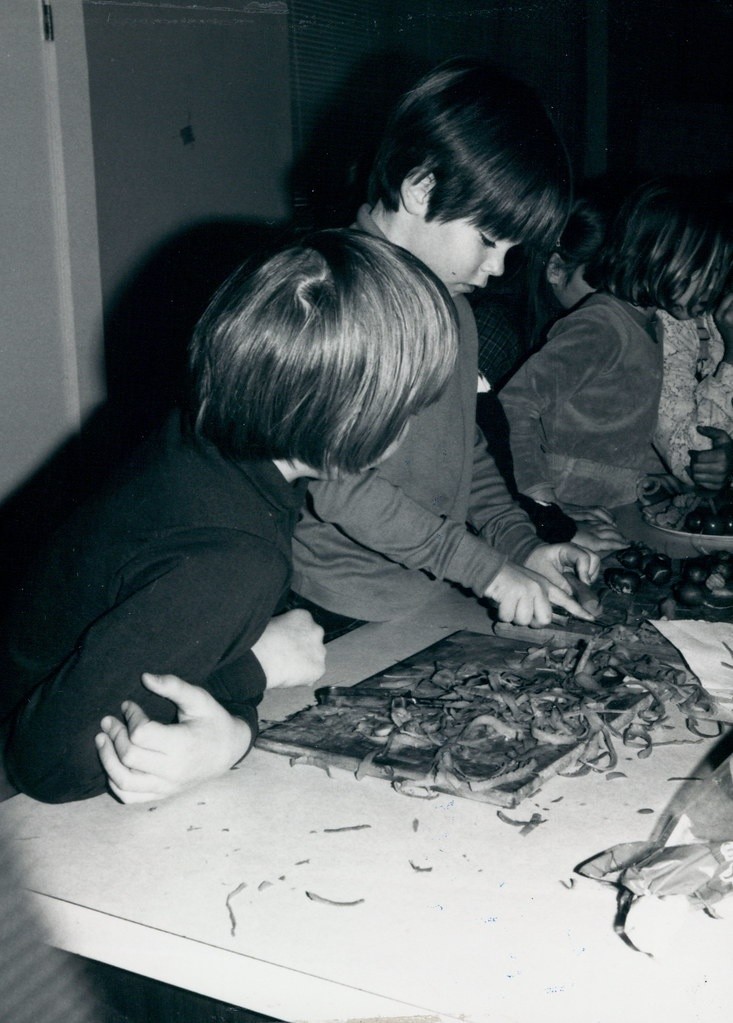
[642,515,733,544]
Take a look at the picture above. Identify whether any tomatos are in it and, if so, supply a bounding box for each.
[606,509,733,609]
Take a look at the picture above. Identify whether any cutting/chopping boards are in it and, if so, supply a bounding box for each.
[493,545,733,664]
[254,630,660,809]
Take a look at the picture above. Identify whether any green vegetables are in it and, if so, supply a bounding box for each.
[220,623,733,937]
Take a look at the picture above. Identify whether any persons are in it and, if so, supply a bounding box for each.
[0,227,461,805]
[287,55,601,644]
[496,174,733,524]
[471,197,609,402]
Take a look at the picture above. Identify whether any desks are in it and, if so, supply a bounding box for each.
[0,581,716,1023]
